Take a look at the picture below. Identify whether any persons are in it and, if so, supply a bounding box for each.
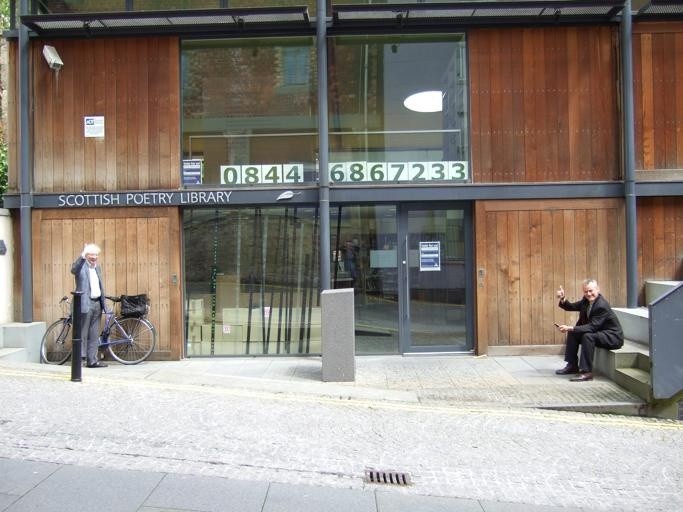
[546,277,624,382]
[71,243,111,367]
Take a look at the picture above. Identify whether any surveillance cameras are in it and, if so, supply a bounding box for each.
[41,43,64,72]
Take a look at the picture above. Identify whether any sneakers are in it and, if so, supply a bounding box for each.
[86,360,110,369]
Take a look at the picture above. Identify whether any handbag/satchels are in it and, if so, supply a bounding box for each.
[119,291,151,319]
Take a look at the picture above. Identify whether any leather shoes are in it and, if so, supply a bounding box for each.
[569,370,594,383]
[554,363,579,376]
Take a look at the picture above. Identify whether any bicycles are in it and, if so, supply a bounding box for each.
[41,296,156,365]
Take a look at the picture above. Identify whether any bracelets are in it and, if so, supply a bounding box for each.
[560,296,566,300]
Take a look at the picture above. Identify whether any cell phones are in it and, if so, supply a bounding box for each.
[553,321,561,329]
